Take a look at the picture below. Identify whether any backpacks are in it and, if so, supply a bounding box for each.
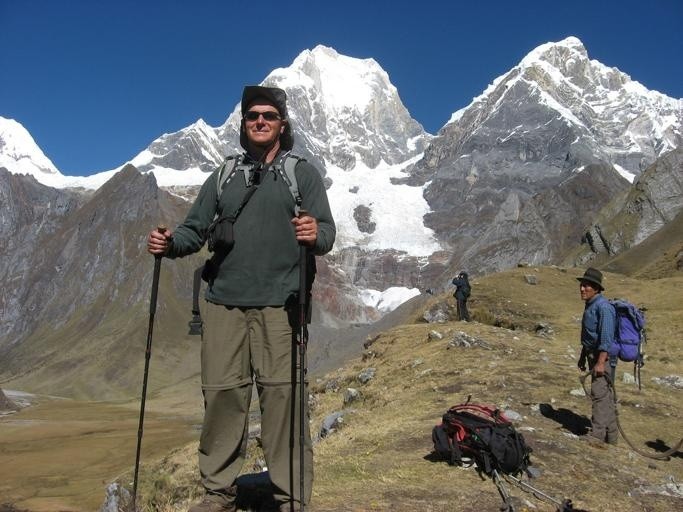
[593,296,644,363]
[429,402,534,479]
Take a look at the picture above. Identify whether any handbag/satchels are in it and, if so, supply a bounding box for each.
[205,213,236,256]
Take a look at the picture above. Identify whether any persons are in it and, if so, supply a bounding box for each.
[575,267,620,447]
[146,84,337,512]
[451,271,471,322]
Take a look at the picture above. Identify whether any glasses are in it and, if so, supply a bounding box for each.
[241,108,283,122]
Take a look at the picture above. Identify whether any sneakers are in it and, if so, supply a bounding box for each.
[277,500,302,512]
[186,492,238,511]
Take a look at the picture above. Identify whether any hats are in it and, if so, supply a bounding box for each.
[235,84,295,151]
[573,268,608,294]
